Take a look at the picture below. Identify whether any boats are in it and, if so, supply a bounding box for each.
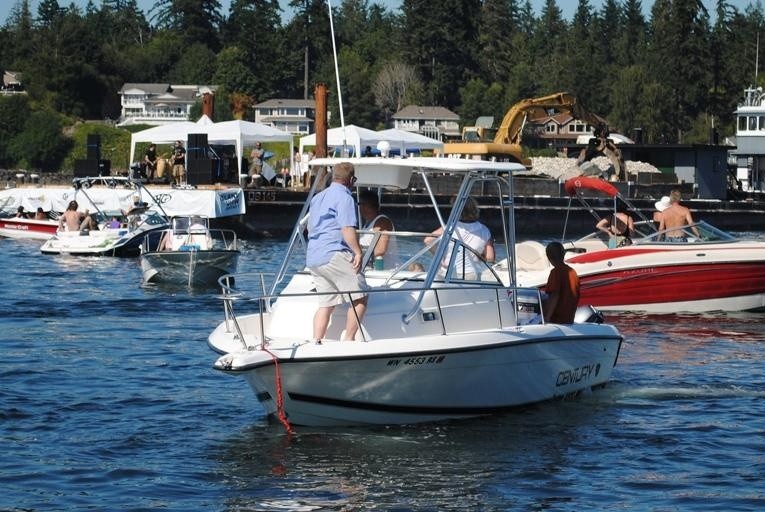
[210,284,627,431]
[480,175,765,316]
[0,176,238,287]
[205,155,547,356]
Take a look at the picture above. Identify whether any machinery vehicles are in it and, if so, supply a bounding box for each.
[434,92,631,181]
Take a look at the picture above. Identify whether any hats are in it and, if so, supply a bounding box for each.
[654,196,673,212]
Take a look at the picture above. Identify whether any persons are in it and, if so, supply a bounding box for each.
[595,198,634,248]
[144,144,159,179]
[170,141,186,185]
[245,141,375,189]
[15,206,26,218]
[648,195,672,242]
[422,195,496,282]
[656,189,700,243]
[359,216,393,269]
[408,263,424,272]
[57,195,149,232]
[304,162,371,341]
[540,241,581,324]
[25,207,45,220]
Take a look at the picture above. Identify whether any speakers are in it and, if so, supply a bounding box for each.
[187,133,224,184]
[73,134,111,177]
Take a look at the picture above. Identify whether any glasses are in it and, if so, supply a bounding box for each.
[352,175,358,182]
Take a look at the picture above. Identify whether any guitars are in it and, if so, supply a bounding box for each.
[167,150,179,168]
[149,151,169,169]
